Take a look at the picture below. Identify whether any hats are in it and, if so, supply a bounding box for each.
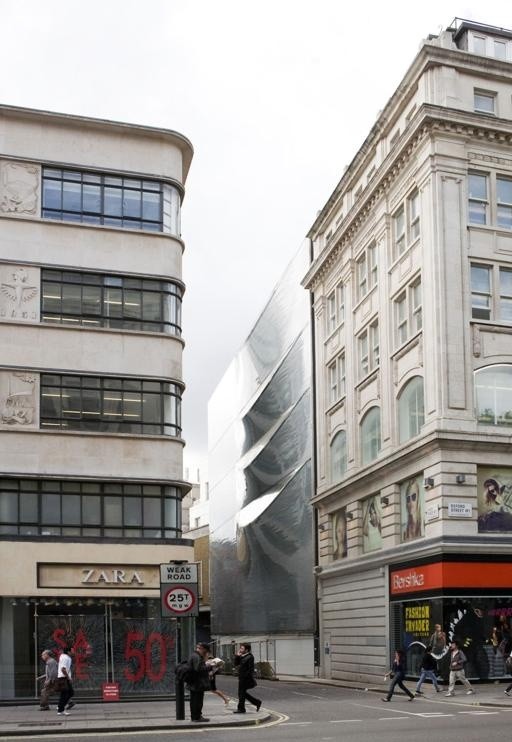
[198,641,212,654]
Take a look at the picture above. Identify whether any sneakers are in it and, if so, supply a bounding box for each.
[57,710,71,716]
[66,702,75,709]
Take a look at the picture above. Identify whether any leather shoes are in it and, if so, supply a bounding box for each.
[192,715,210,722]
[256,701,261,712]
[233,709,243,713]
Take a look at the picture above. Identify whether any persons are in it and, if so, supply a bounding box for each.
[234,643,261,714]
[196,642,230,707]
[479,475,512,531]
[428,623,447,655]
[333,512,347,560]
[361,499,382,551]
[179,644,212,722]
[57,647,74,716]
[416,648,443,695]
[505,649,512,694]
[36,650,74,711]
[382,650,414,702]
[402,478,425,538]
[445,642,475,696]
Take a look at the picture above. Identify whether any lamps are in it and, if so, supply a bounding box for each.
[380,496,389,511]
[344,509,355,523]
[455,472,466,485]
[423,475,436,490]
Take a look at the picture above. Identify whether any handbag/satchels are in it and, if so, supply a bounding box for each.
[173,660,192,682]
[54,676,68,692]
